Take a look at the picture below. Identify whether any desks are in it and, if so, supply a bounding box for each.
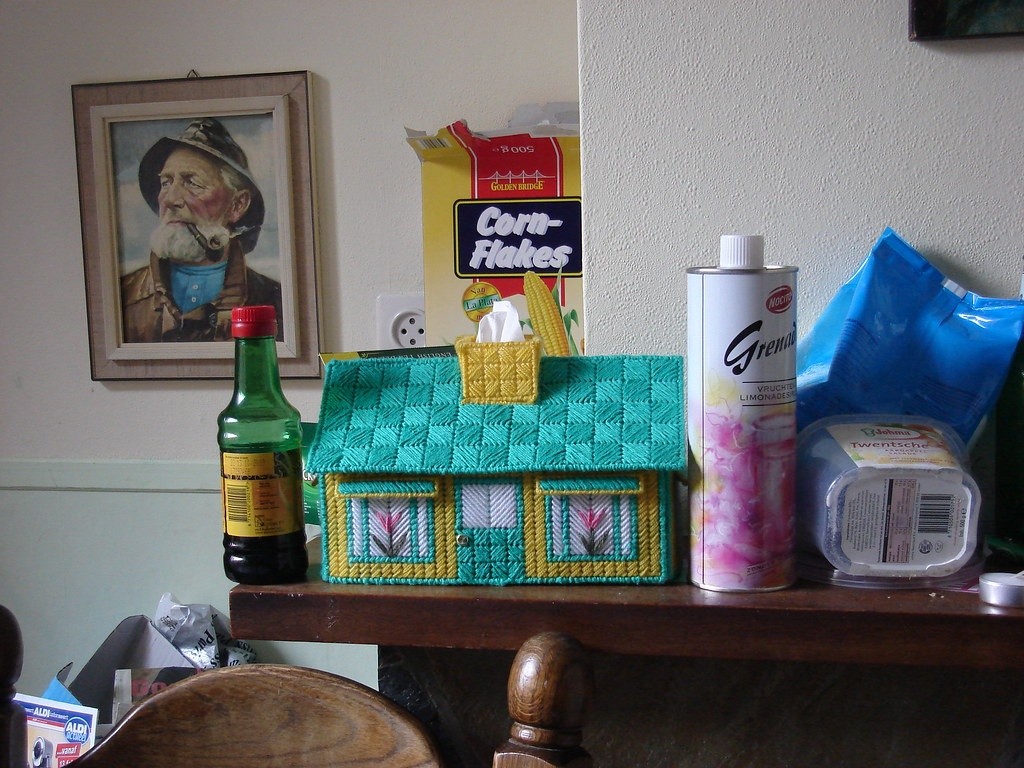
[229,528,1024,768]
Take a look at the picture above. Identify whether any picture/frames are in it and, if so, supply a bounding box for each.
[69,70,328,382]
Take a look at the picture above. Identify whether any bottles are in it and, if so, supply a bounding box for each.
[218,305,310,583]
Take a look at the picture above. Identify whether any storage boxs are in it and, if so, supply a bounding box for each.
[796,414,987,581]
[402,100,585,355]
